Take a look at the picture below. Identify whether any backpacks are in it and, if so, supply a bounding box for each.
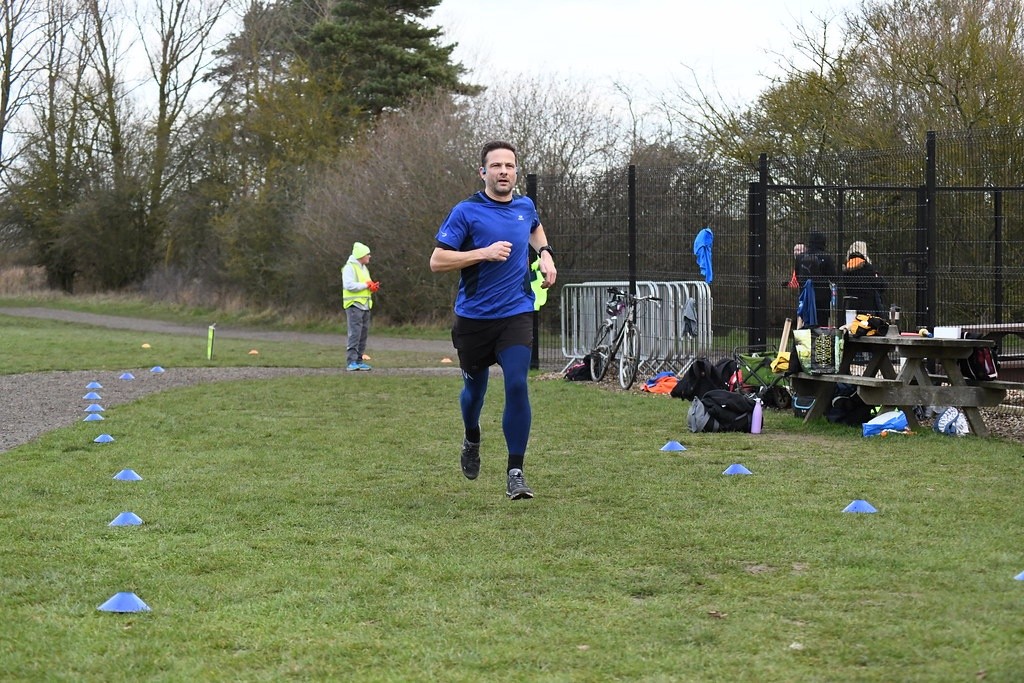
[680,357,750,399]
[958,331,1001,380]
[566,363,599,381]
[824,381,874,422]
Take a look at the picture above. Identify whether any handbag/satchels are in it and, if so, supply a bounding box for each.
[686,396,763,432]
[791,396,818,416]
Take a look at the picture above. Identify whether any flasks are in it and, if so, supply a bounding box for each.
[845,309,857,329]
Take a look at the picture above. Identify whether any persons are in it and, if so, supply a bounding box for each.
[526,249,548,371]
[784,241,812,332]
[427,141,559,501]
[796,230,841,330]
[342,241,381,371]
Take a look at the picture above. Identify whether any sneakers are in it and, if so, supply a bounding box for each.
[346,361,359,371]
[358,364,371,370]
[506,468,533,500]
[461,424,481,479]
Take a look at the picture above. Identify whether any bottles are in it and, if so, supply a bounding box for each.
[752,397,763,434]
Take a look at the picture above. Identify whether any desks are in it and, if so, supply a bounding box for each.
[783,336,1024,437]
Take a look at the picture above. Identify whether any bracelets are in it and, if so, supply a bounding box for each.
[538,245,554,258]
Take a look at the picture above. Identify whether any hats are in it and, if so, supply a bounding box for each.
[846,241,872,266]
[352,242,370,260]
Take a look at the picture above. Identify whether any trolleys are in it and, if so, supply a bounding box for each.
[733,344,794,409]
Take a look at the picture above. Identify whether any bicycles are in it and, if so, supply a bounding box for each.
[590,287,663,390]
